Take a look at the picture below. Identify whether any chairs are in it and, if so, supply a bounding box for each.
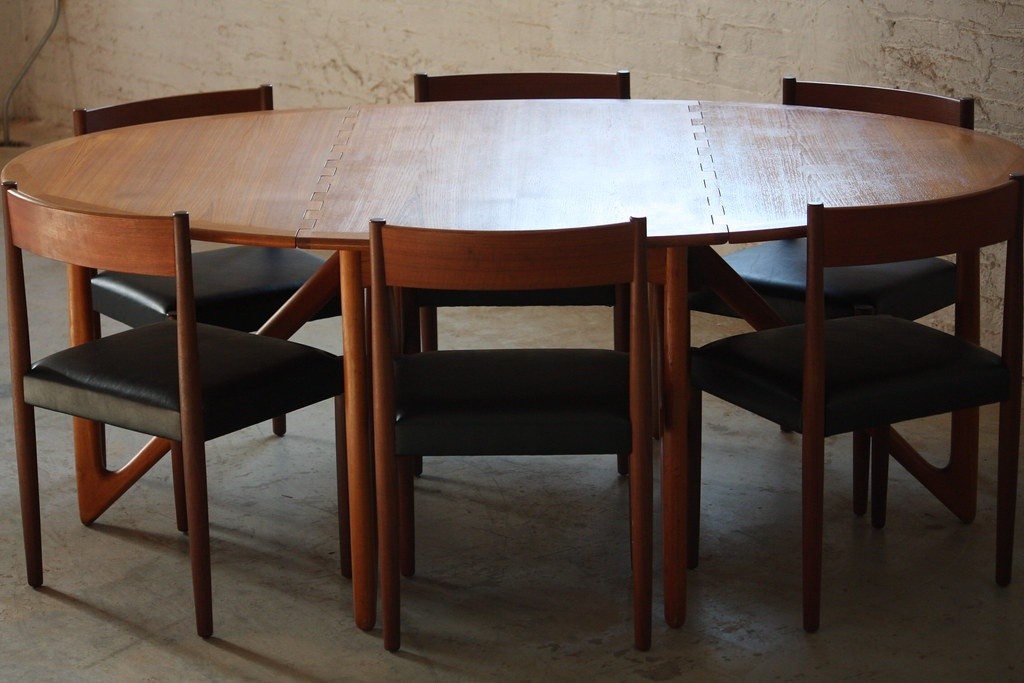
[412,71,632,479]
[0,178,354,639]
[689,74,975,493]
[370,216,664,653]
[699,173,1024,631]
[74,83,341,436]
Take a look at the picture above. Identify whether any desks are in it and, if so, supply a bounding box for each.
[0,98,1024,635]
